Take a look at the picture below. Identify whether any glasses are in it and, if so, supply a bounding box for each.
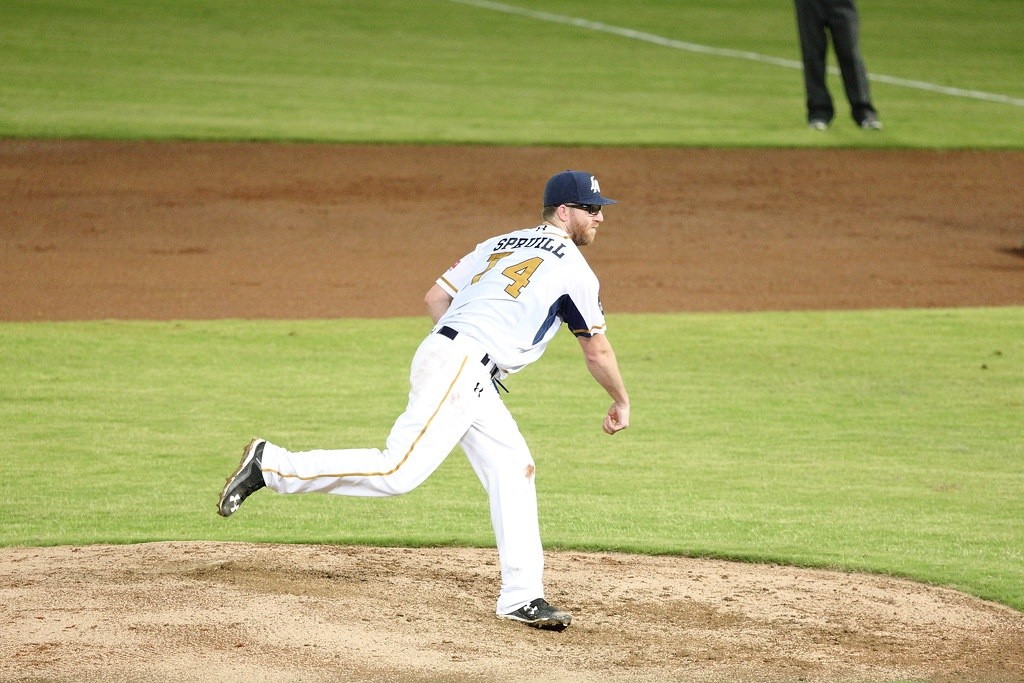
[565,202,601,214]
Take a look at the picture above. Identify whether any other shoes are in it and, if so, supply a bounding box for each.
[862,117,882,128]
[810,120,827,130]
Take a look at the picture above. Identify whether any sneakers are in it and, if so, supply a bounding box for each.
[216,436,266,518]
[497,598,571,631]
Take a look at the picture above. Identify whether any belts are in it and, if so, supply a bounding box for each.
[436,324,502,377]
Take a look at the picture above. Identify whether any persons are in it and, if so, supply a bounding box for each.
[217,169,631,631]
[795,0,884,132]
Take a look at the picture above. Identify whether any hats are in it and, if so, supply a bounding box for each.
[544,168,613,208]
